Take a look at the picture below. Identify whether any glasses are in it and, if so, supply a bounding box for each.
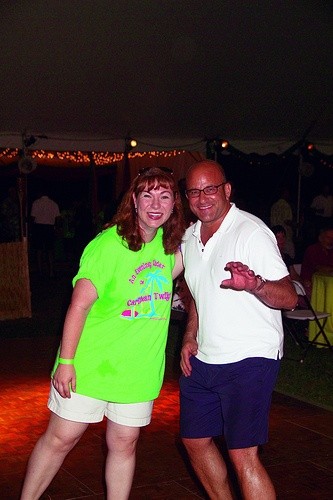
[137,167,176,182]
[185,183,226,198]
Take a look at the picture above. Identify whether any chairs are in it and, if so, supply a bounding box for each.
[281,279,333,364]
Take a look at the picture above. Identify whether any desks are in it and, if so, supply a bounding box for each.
[308,272,333,348]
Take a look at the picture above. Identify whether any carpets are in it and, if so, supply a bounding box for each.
[166,325,333,411]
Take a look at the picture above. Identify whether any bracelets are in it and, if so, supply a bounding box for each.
[58,358,74,365]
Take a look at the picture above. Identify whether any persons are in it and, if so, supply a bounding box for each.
[20,166,194,500]
[29,184,333,312]
[178,159,299,500]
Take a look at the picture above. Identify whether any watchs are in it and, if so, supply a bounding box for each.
[252,275,266,293]
